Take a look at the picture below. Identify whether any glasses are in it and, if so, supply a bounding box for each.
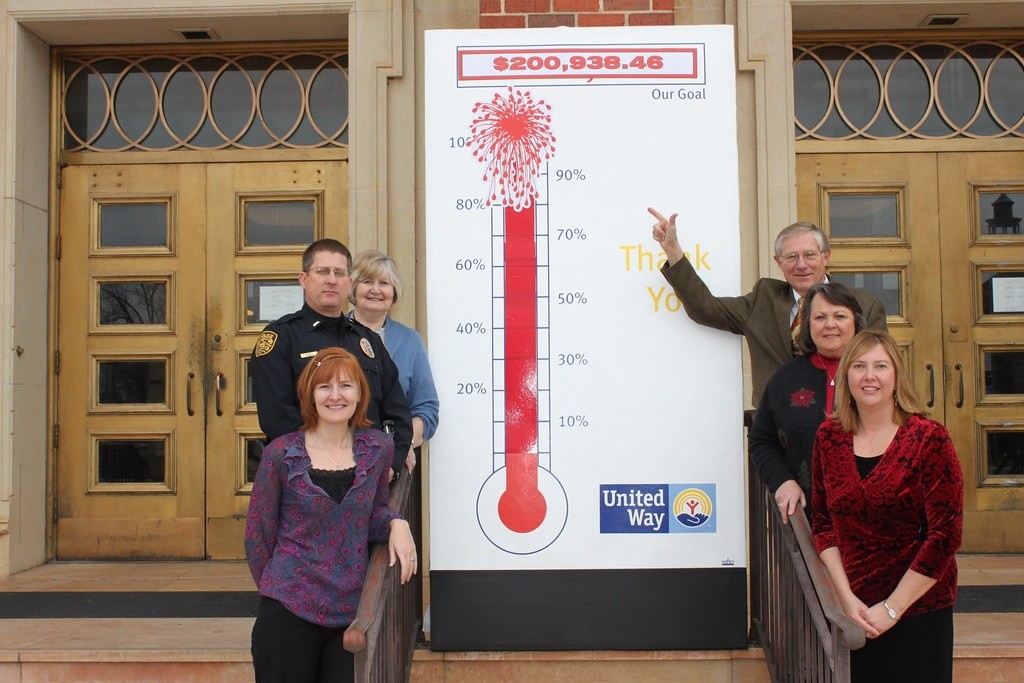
[305,267,347,277]
[779,249,826,263]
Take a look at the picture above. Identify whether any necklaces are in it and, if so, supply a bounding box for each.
[817,352,837,386]
[313,434,346,468]
[378,328,383,334]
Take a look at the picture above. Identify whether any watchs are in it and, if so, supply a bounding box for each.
[884,601,900,620]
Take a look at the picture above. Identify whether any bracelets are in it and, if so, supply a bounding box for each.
[412,440,414,448]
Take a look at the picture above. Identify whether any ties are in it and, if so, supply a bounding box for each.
[789,297,805,360]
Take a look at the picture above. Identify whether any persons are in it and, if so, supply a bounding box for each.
[752,284,865,525]
[809,329,964,682]
[648,207,889,408]
[344,249,440,475]
[250,238,413,489]
[244,348,417,683]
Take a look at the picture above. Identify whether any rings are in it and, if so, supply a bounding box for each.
[410,559,415,562]
[778,504,782,506]
